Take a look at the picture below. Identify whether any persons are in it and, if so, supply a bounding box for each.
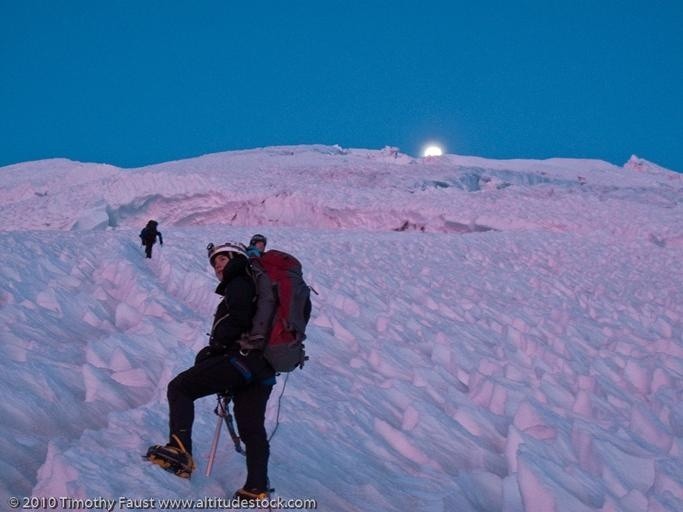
[147,241,276,505]
[139,220,163,258]
[246,234,266,257]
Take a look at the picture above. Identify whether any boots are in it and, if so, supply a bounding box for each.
[235,476,275,505]
[146,424,194,481]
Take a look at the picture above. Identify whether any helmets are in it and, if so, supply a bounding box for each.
[250,234,267,247]
[207,240,249,266]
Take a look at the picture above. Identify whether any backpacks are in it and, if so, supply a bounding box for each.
[249,247,320,374]
